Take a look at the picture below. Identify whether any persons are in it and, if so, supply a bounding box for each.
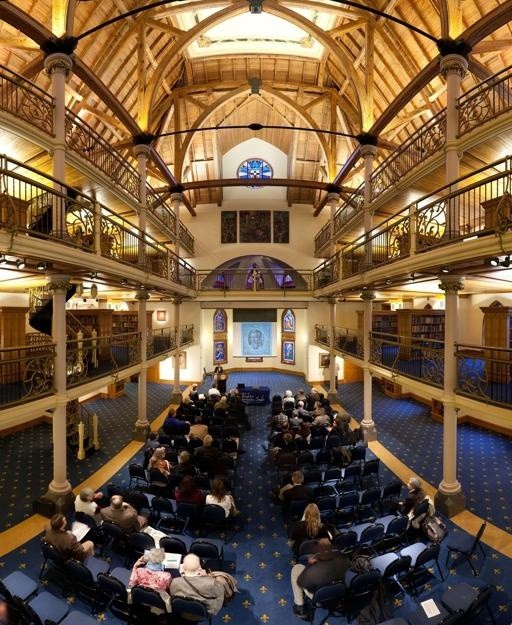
[45,488,229,615]
[146,382,248,528]
[267,387,352,472]
[279,471,428,616]
[214,363,223,373]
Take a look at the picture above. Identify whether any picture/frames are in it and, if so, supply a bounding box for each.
[157,311,166,321]
[319,353,336,368]
[172,351,186,369]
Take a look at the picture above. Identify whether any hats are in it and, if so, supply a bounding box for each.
[183,553,200,576]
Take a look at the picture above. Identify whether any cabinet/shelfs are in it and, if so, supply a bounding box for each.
[398,312,445,361]
[112,315,152,347]
[64,314,112,360]
[358,314,397,349]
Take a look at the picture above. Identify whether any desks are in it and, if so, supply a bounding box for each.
[246,356,263,362]
[236,386,271,406]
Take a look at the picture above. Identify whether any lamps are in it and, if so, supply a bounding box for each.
[0,255,145,291]
[362,255,512,290]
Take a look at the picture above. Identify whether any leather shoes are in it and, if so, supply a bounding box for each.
[293,606,306,618]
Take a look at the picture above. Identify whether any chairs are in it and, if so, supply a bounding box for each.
[0,392,496,625]
